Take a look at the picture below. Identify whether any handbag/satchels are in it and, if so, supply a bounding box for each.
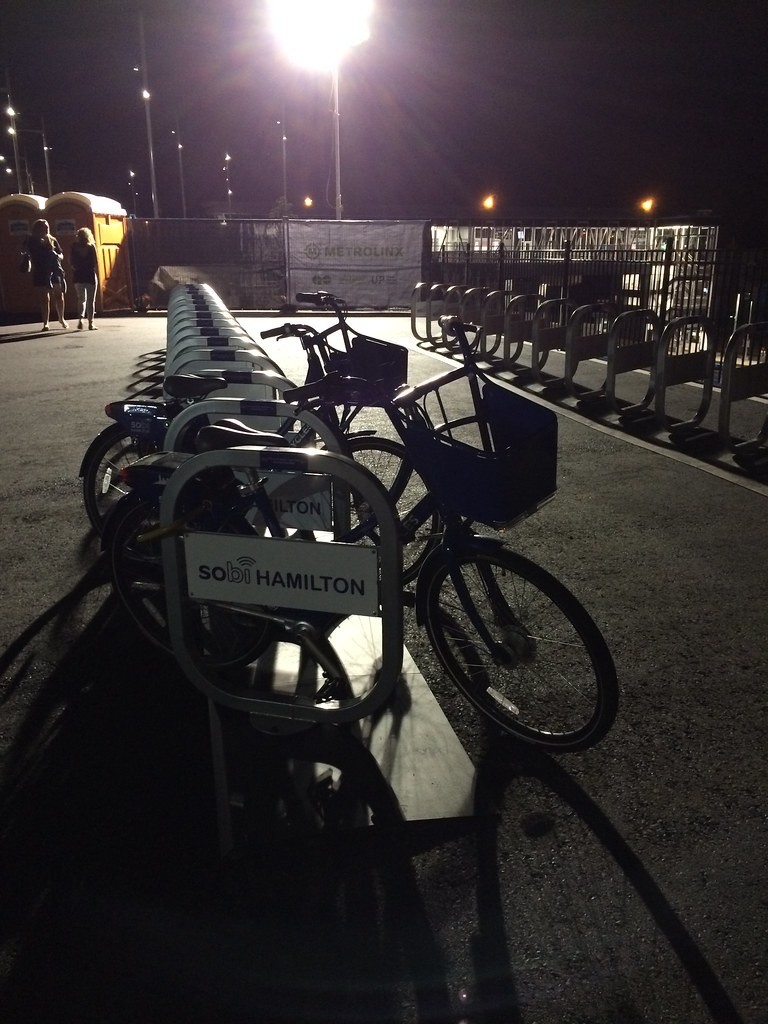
[17,254,31,272]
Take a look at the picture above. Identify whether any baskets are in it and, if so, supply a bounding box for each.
[392,378,557,528]
[326,337,410,408]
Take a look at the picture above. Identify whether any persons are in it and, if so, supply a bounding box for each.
[22,219,67,332]
[69,228,98,330]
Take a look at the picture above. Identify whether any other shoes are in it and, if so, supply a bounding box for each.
[78,322,82,329]
[59,319,67,328]
[42,327,48,331]
[89,321,93,330]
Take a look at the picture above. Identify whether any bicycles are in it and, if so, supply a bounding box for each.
[78,288,443,594]
[108,306,624,753]
